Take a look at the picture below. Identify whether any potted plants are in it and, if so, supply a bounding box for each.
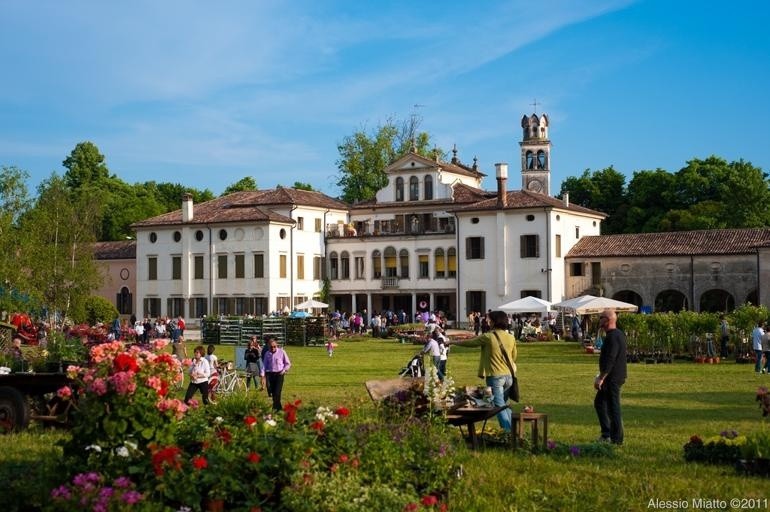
[614,300,770,364]
[43,325,86,374]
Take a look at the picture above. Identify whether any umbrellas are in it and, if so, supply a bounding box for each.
[494,294,639,335]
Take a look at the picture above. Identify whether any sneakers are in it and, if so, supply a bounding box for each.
[590,435,610,444]
[720,354,729,361]
[753,365,770,376]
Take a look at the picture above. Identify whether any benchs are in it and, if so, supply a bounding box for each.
[365,375,509,443]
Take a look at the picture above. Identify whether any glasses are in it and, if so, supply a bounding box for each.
[598,315,608,320]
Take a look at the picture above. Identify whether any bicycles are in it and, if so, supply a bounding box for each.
[206,358,249,404]
[169,353,184,391]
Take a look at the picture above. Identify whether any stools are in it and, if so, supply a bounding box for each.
[511,412,548,455]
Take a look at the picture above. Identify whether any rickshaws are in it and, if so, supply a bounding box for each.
[362,371,515,451]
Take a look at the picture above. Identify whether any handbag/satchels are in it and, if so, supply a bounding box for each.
[249,362,258,372]
[508,374,521,403]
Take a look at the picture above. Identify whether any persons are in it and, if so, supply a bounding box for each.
[593,310,627,447]
[411,215,419,234]
[1,311,451,412]
[761,326,770,375]
[348,220,358,237]
[752,319,766,373]
[445,311,525,441]
[367,216,374,237]
[718,315,730,360]
[468,308,607,350]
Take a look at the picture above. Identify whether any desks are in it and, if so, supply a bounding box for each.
[447,403,495,452]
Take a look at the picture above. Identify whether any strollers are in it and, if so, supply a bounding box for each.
[399,351,425,379]
